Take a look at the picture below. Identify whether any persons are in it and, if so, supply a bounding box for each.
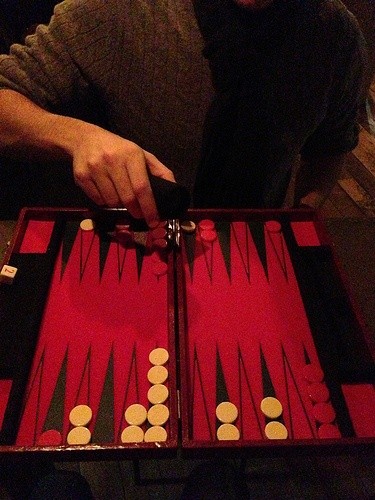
[0,0,367,228]
